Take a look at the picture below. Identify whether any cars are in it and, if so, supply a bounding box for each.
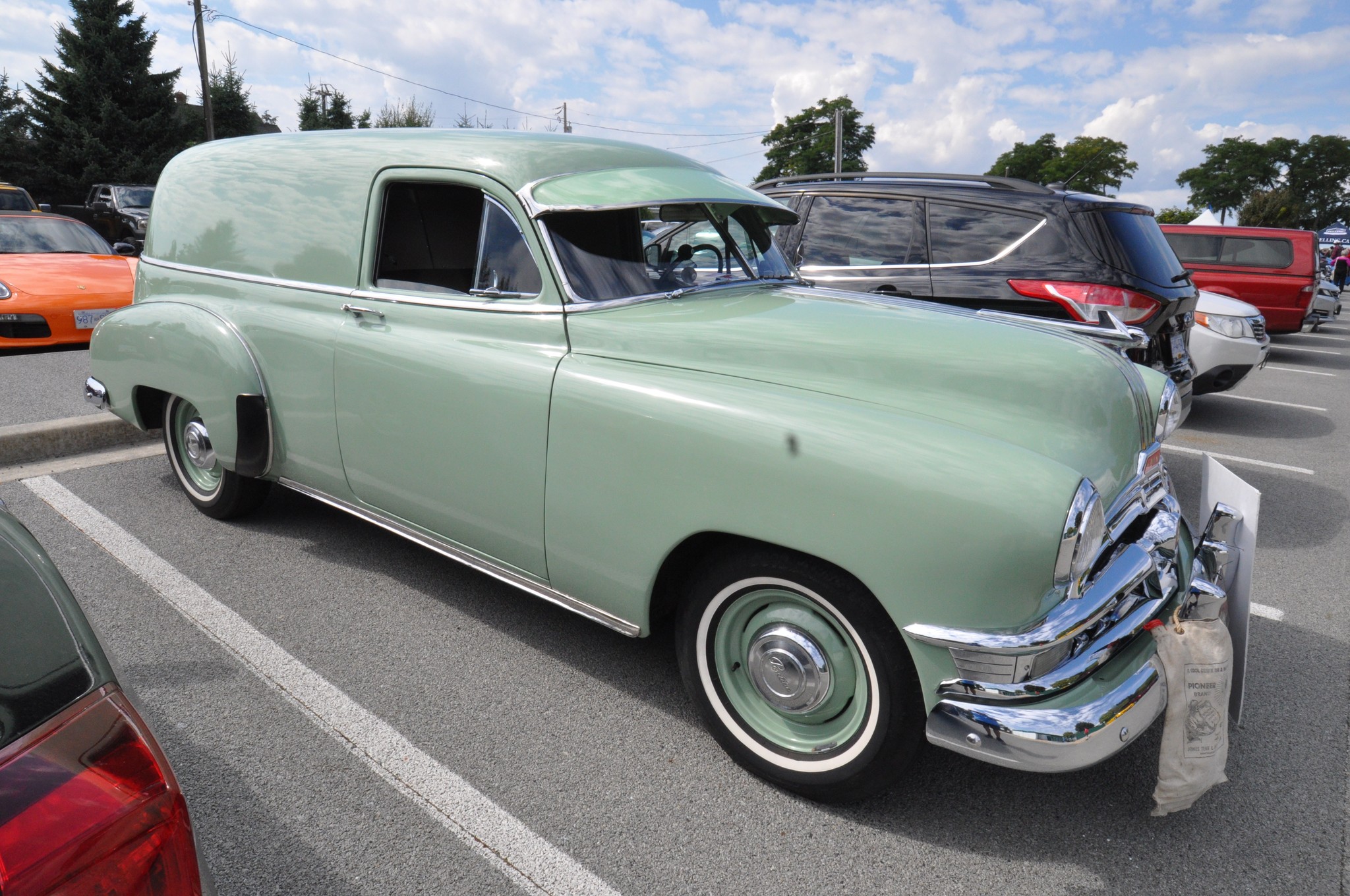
[80,125,1244,808]
[1313,278,1344,324]
[1,495,203,895]
[0,181,54,213]
[0,209,140,353]
[1188,289,1272,398]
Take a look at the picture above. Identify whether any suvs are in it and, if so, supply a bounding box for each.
[655,168,1200,433]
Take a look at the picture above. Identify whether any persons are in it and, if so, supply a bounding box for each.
[1319,239,1350,294]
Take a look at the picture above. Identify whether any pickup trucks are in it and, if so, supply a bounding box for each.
[37,182,157,251]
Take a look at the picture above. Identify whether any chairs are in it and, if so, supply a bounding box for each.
[801,208,851,267]
[880,212,926,265]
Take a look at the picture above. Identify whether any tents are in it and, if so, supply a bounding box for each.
[1187,209,1223,226]
[1316,221,1350,248]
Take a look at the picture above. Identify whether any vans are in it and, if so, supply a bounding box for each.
[1158,223,1321,334]
